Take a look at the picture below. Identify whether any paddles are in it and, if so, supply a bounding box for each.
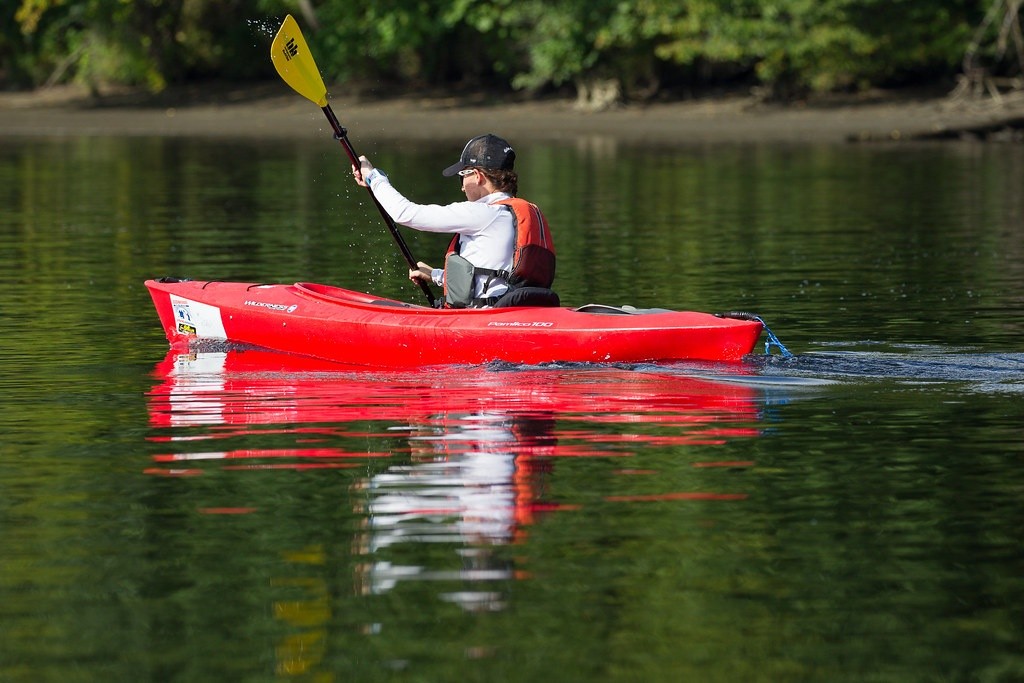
[269,13,438,310]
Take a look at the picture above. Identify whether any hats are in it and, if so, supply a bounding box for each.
[442,133,516,176]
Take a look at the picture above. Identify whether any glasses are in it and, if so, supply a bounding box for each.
[456,169,487,186]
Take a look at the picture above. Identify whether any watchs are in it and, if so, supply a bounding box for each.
[366,168,387,185]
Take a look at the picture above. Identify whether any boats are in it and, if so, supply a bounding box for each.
[144,276,798,371]
[146,341,768,429]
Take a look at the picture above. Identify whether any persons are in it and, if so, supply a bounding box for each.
[352,135,556,306]
